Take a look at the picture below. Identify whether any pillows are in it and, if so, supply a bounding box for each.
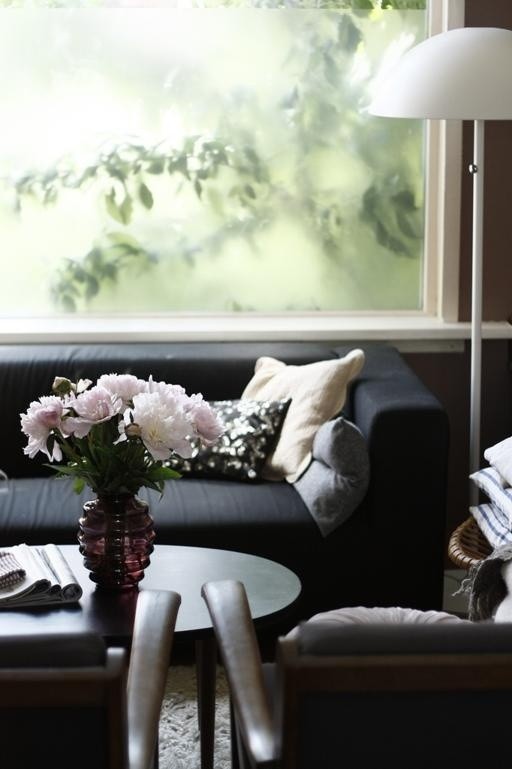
[239,350,365,484]
[469,436,512,623]
[161,398,291,484]
[292,417,370,538]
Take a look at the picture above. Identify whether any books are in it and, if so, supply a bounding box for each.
[0,542,83,607]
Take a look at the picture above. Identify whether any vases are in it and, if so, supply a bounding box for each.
[78,494,156,587]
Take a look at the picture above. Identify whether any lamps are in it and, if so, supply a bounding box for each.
[368,26,512,506]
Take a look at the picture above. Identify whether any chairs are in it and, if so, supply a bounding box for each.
[0,591,180,769]
[203,580,512,769]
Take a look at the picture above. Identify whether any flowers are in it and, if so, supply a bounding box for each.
[21,375,224,578]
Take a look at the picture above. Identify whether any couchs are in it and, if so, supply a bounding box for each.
[0,341,450,611]
